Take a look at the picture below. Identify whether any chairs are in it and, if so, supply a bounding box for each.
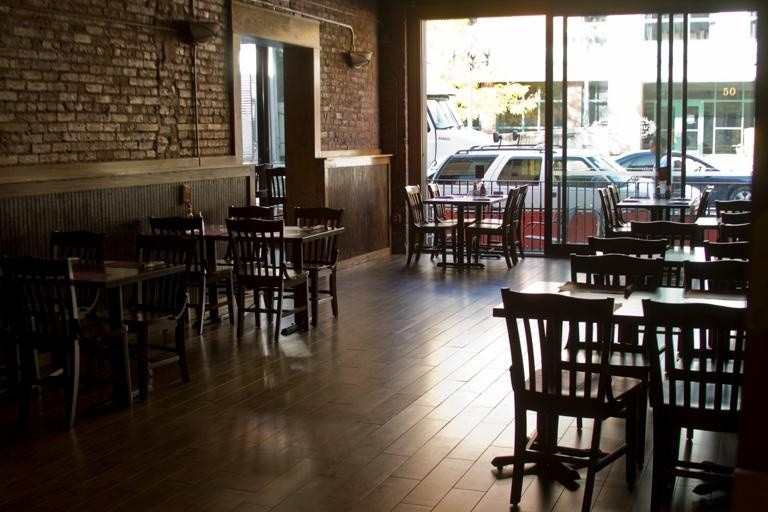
[143,197,345,345]
[492,255,755,512]
[0,224,194,428]
[265,168,289,215]
[588,177,754,289]
[401,178,529,270]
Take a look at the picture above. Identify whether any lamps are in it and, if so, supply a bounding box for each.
[181,17,221,46]
[347,47,374,69]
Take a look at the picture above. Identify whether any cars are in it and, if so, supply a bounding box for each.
[614,150,754,210]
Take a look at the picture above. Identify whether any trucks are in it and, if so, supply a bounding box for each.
[426,88,493,176]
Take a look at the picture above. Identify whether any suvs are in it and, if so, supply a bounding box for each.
[428,130,737,251]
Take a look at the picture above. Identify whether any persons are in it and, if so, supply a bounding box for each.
[647,135,674,199]
